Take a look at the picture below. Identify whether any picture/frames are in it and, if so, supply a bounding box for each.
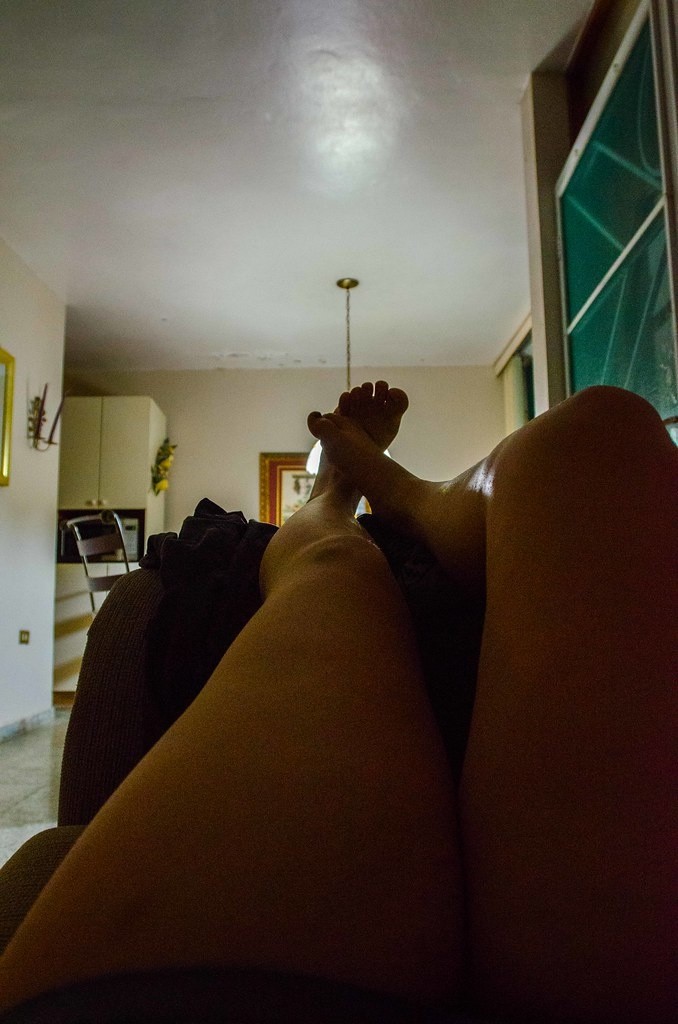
[258,452,372,528]
[0,345,15,487]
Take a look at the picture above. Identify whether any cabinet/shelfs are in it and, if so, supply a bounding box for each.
[59,395,167,510]
[54,562,141,693]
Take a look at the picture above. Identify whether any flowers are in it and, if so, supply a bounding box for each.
[150,435,177,496]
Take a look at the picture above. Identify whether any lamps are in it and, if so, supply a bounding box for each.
[305,277,391,476]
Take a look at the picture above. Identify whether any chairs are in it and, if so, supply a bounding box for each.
[66,508,130,619]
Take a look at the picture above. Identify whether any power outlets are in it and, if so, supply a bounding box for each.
[19,630,29,644]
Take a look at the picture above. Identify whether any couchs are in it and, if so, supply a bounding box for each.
[0,568,485,956]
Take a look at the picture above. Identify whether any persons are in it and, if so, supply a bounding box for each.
[0,381,678,1024]
